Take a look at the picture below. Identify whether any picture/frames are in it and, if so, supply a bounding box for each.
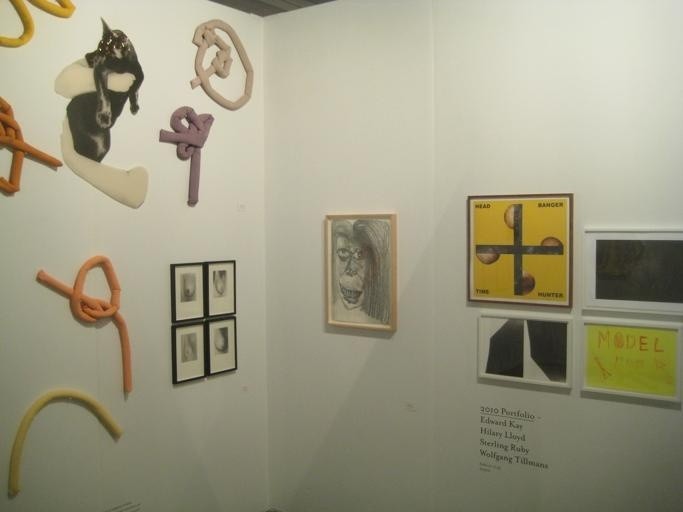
[205,259,237,318]
[579,226,683,317]
[475,312,574,392]
[206,317,237,377]
[323,213,398,333]
[170,321,207,385]
[578,318,682,405]
[465,192,573,309]
[168,261,206,323]
